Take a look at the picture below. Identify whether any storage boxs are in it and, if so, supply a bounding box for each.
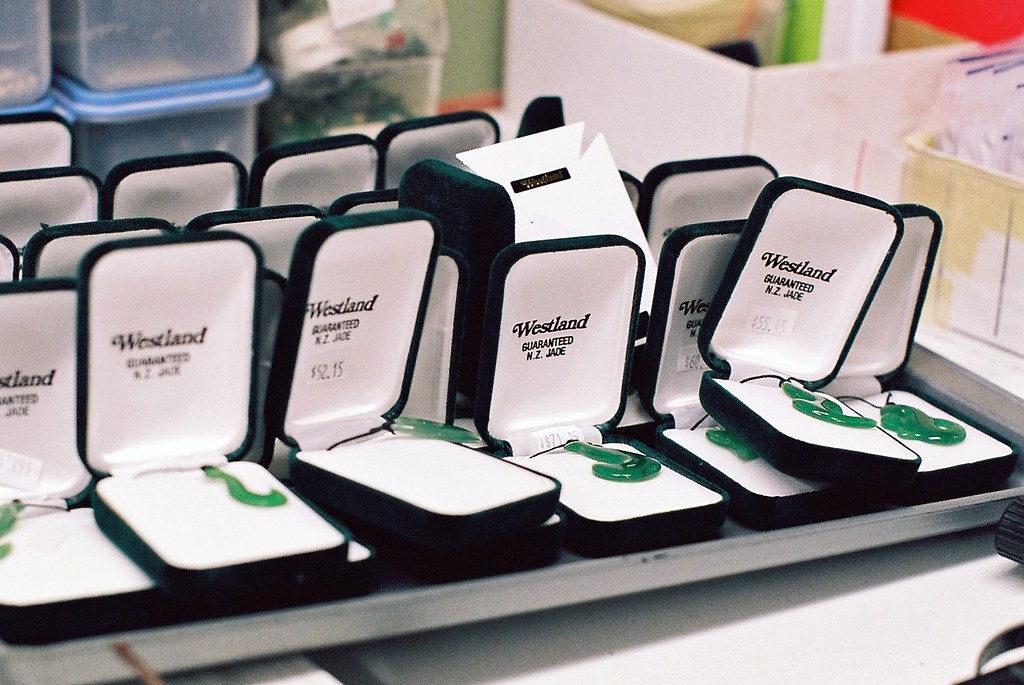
[0,0,1024,650]
